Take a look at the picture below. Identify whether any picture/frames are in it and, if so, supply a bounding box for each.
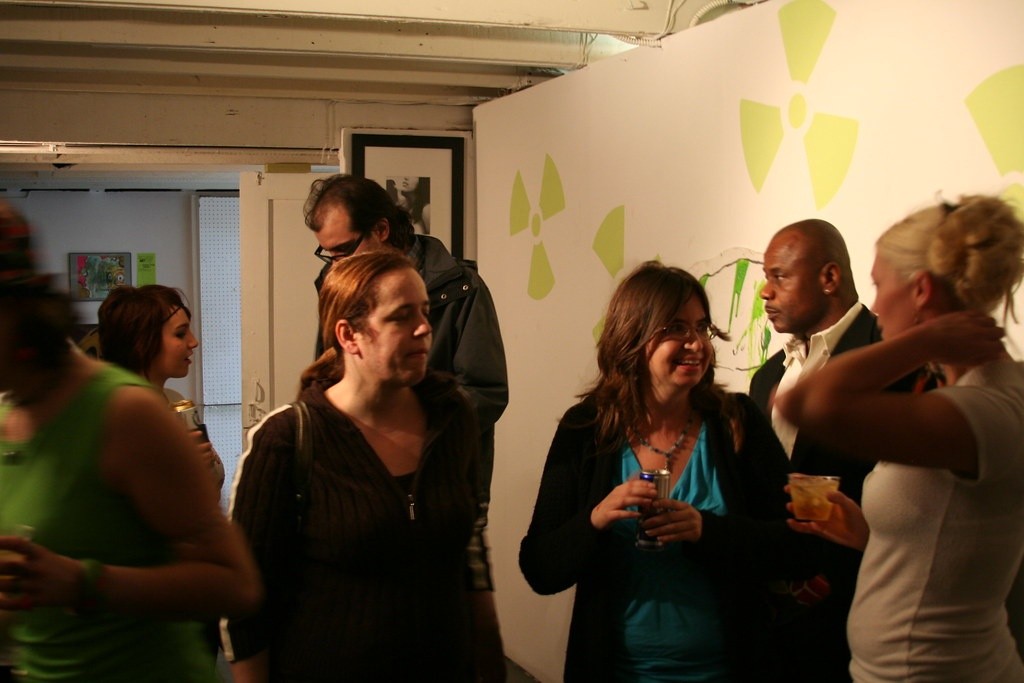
[67,251,134,303]
[350,132,465,259]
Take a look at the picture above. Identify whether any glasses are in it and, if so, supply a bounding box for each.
[660,322,717,341]
[315,227,369,266]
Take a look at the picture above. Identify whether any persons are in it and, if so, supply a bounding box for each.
[782,195,1024,683]
[304,175,510,592]
[0,204,260,683]
[216,249,483,683]
[520,260,769,683]
[398,177,431,235]
[97,284,226,502]
[749,219,915,683]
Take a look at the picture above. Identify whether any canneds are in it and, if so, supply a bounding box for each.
[171,398,202,430]
[635,468,671,552]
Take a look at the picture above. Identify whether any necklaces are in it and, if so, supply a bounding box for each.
[632,409,694,472]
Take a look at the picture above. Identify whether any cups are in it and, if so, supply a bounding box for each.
[788,475,840,521]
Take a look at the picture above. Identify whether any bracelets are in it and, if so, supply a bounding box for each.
[78,559,101,604]
